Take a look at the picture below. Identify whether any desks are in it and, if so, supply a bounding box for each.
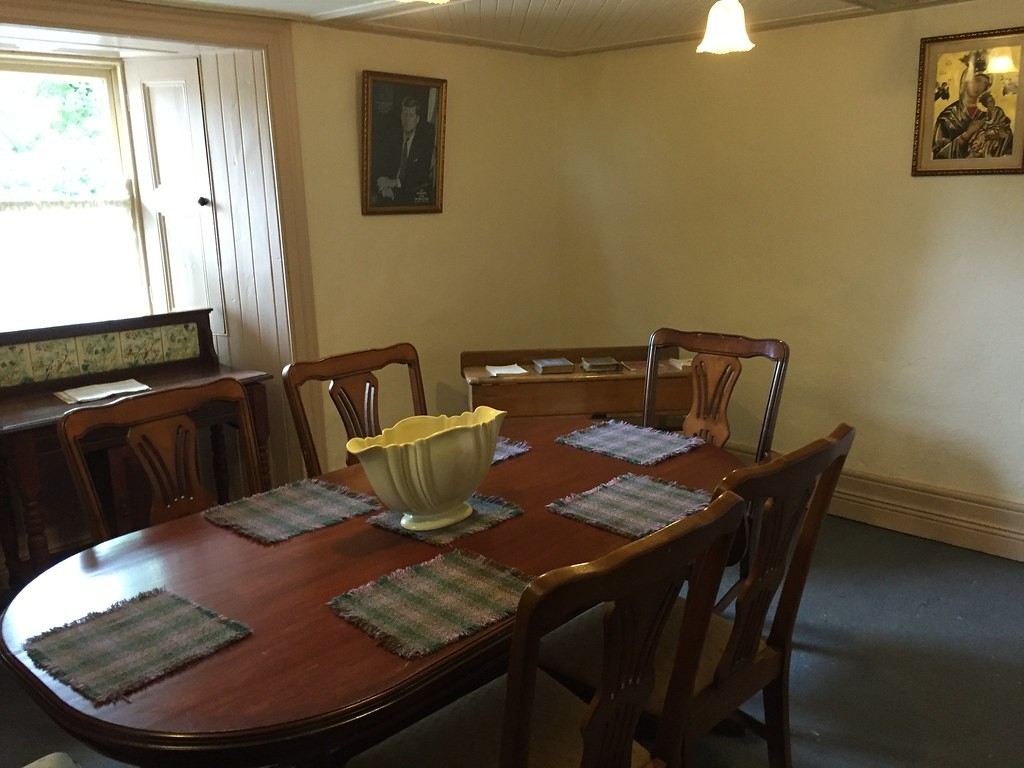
[0,308,276,595]
[459,342,695,427]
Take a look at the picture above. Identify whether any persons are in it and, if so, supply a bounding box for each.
[378,95,434,206]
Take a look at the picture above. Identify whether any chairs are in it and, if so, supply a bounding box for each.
[55,378,265,546]
[534,423,855,768]
[281,342,428,478]
[342,491,747,768]
[641,327,789,463]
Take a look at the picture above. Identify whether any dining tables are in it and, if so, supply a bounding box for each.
[0,428,747,768]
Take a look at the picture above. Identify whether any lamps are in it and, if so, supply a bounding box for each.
[695,0,756,54]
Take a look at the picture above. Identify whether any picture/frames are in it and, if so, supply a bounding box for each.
[360,69,448,215]
[910,26,1024,177]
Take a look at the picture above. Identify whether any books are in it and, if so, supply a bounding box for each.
[531,355,576,375]
[670,355,703,372]
[580,356,621,373]
[622,360,667,372]
